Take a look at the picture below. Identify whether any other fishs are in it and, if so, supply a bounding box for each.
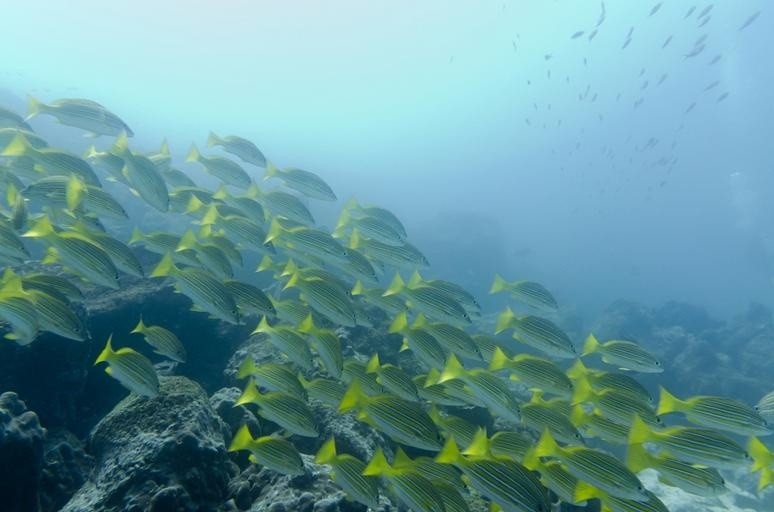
[0,92,774,512]
